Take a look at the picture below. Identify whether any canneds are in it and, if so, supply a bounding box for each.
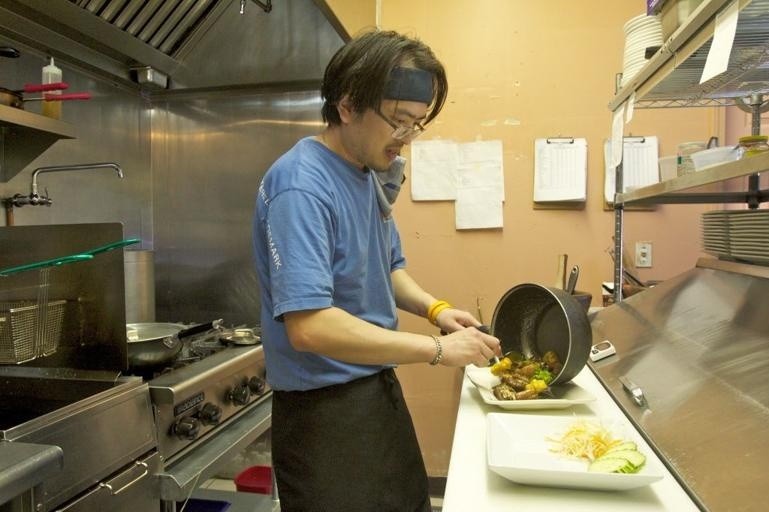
[736,135,769,161]
[677,141,706,176]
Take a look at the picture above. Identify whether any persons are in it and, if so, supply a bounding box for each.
[251,26,499,511]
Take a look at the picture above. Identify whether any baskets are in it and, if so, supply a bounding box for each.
[0,299,67,365]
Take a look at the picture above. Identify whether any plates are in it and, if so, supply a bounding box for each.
[467,365,597,412]
[479,412,665,492]
[729,209,768,265]
[698,209,728,260]
[619,14,665,90]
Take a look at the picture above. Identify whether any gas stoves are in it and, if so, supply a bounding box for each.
[133,321,262,405]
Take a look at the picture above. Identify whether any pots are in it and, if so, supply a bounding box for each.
[1,86,91,111]
[439,283,593,388]
[127,317,223,365]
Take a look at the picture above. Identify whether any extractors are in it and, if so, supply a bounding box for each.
[0,0,355,103]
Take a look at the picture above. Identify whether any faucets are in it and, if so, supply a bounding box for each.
[29,161,125,206]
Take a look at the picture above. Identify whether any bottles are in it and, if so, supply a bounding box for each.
[41,57,64,122]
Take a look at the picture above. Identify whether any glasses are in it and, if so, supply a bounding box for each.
[365,105,425,140]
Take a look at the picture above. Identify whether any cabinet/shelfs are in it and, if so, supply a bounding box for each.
[596,0,769,303]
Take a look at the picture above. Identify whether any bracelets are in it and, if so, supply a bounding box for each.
[427,300,447,324]
[430,334,442,365]
[432,303,452,325]
